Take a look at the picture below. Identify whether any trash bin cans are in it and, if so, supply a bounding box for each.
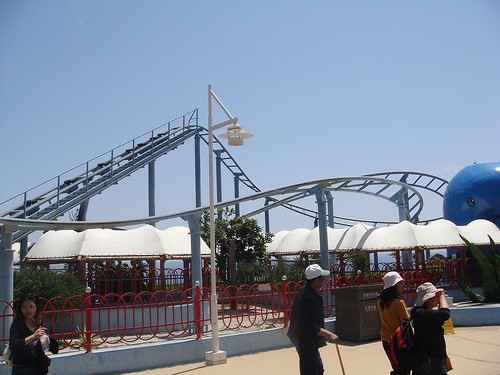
[334,284,384,342]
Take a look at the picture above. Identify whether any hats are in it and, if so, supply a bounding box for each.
[382,271,404,289]
[414,282,438,306]
[305,264,331,280]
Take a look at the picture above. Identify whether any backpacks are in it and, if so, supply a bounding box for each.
[390,309,423,369]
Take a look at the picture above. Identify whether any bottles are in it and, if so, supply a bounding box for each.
[40,331,49,353]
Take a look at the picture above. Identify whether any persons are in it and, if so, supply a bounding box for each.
[9,294,51,375]
[376,271,414,375]
[287,264,339,375]
[409,281,451,375]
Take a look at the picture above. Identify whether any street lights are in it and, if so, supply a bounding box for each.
[203,81,254,366]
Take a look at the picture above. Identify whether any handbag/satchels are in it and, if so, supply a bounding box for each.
[3,346,14,367]
